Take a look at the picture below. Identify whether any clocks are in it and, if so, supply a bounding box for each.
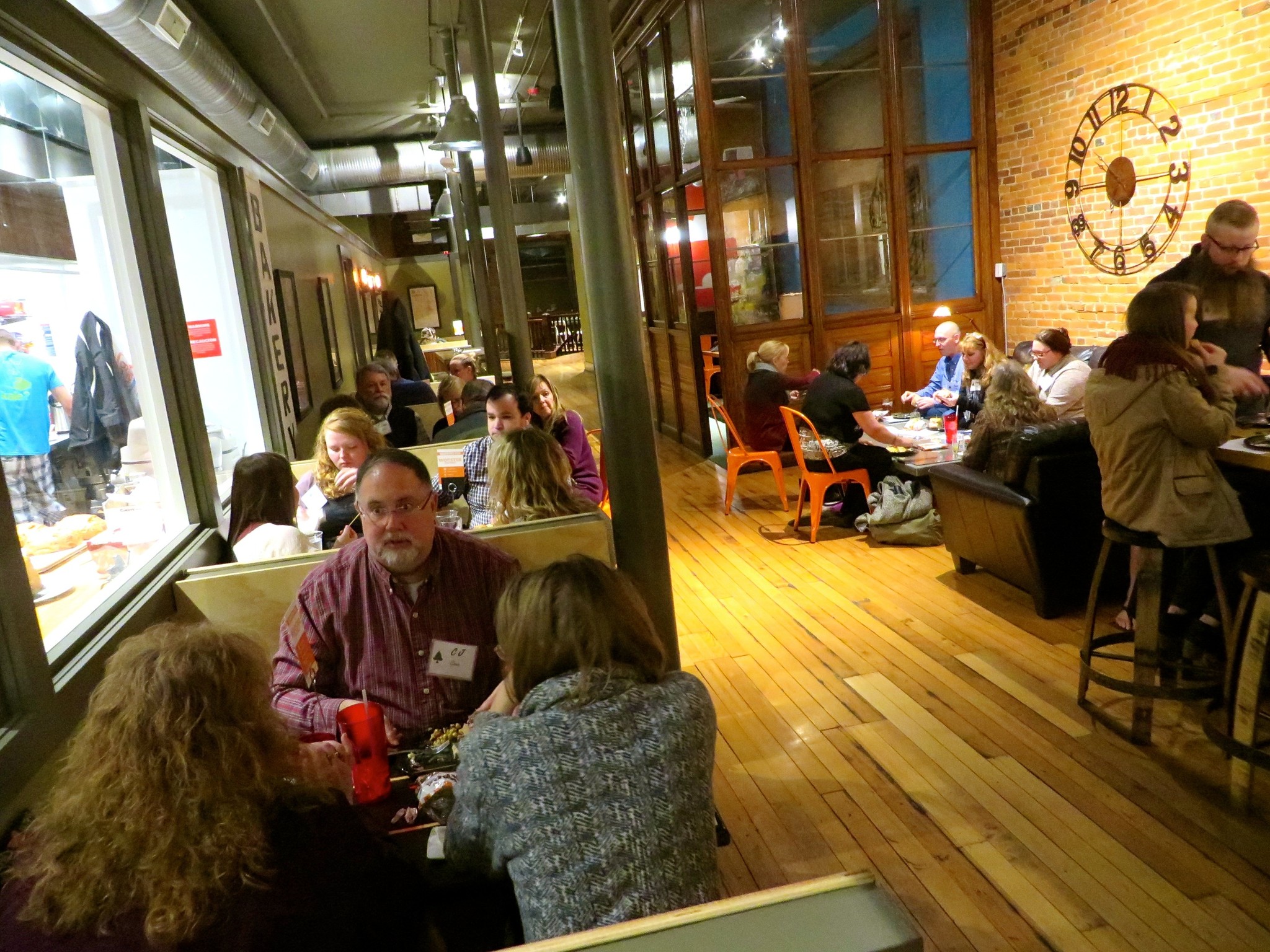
[1063,83,1192,274]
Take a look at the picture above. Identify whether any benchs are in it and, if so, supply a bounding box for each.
[289,438,486,513]
[173,513,610,682]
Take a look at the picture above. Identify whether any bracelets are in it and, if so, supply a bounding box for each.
[890,435,897,446]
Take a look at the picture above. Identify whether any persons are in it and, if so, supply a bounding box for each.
[429,378,498,445]
[307,407,395,497]
[353,363,420,450]
[431,554,725,941]
[372,349,438,406]
[317,395,359,421]
[897,321,973,420]
[0,311,70,539]
[224,453,327,564]
[1081,280,1241,674]
[432,374,467,439]
[448,358,477,382]
[0,621,425,952]
[792,338,919,525]
[1111,200,1269,634]
[927,332,1016,433]
[493,427,619,572]
[963,361,1059,482]
[461,384,568,528]
[266,446,529,753]
[526,374,605,506]
[1024,328,1093,421]
[741,339,821,454]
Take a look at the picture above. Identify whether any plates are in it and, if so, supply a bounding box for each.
[1234,412,1270,428]
[891,413,911,418]
[886,446,918,456]
[926,426,945,432]
[1244,436,1270,449]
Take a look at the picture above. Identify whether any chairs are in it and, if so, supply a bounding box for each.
[708,395,791,514]
[417,334,467,351]
[779,406,875,543]
[585,429,612,521]
[350,373,496,405]
[704,364,721,404]
[407,402,446,442]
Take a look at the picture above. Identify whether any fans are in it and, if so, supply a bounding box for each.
[738,4,838,75]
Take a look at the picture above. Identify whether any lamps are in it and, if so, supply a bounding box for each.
[515,92,534,167]
[427,0,484,152]
[514,38,524,58]
[433,153,468,220]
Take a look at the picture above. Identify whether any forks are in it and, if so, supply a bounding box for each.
[388,741,450,754]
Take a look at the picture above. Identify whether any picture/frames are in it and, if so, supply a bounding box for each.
[362,289,377,335]
[408,282,442,332]
[376,291,383,321]
[273,267,315,422]
[318,276,344,390]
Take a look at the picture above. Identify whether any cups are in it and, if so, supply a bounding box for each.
[910,412,920,419]
[952,433,964,452]
[880,398,894,416]
[943,415,958,444]
[440,516,463,531]
[434,510,459,525]
[304,530,323,550]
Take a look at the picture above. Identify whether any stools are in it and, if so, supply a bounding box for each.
[1076,515,1238,747]
[1204,562,1270,812]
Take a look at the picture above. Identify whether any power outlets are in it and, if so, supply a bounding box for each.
[995,263,1007,277]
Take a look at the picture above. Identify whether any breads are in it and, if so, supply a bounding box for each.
[16,512,108,555]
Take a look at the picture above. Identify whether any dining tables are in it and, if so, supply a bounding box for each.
[1217,436,1270,475]
[856,411,970,476]
[274,734,732,929]
[703,352,720,358]
[36,541,154,648]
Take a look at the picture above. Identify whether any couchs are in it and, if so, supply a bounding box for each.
[929,337,1133,619]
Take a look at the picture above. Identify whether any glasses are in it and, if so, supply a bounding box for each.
[1207,235,1260,254]
[357,490,434,521]
[932,337,955,343]
[1029,349,1052,357]
